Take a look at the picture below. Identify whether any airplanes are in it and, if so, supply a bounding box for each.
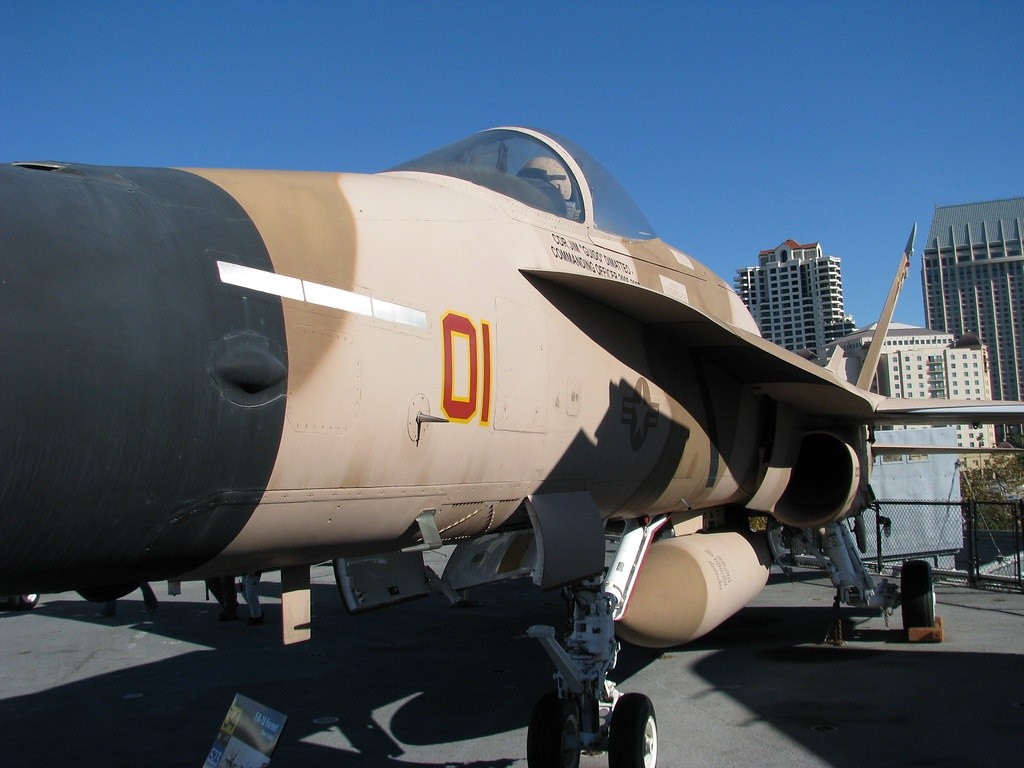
[0,122,1024,767]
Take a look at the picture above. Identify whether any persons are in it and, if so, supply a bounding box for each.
[519,157,582,221]
[95,572,265,626]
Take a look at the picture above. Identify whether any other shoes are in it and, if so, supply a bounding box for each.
[95,611,117,618]
[217,610,240,622]
[141,602,158,614]
